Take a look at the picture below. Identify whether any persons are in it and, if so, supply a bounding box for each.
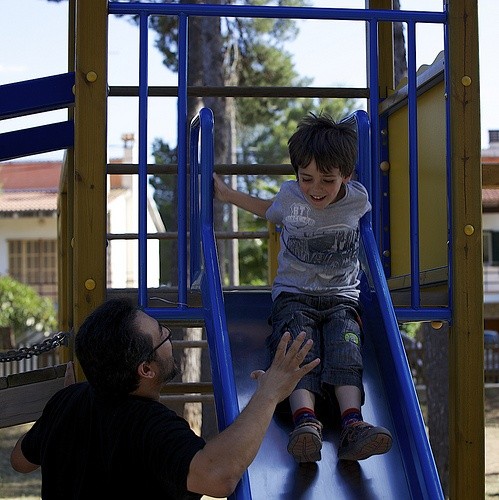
[9,297,321,500]
[213,116,394,463]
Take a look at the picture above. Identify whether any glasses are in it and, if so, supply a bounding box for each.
[142,322,173,360]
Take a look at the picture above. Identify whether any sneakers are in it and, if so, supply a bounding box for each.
[336,416,393,460]
[287,416,326,464]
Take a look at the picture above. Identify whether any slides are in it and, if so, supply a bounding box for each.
[188,108,445,500]
